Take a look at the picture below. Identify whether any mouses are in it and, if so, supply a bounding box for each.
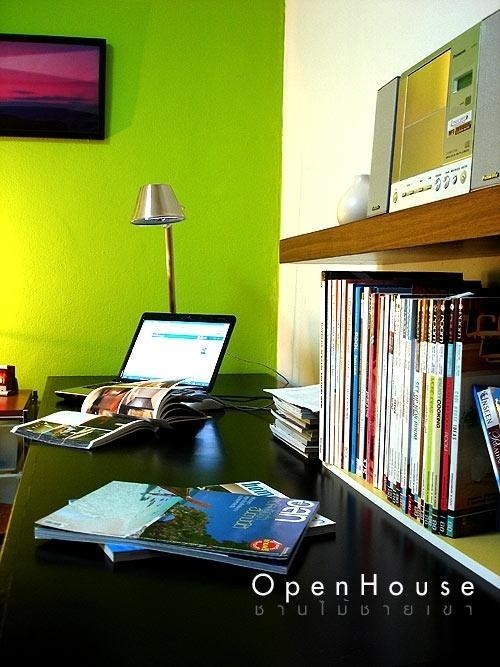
[180,396,226,416]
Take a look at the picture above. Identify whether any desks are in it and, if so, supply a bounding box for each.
[1,371,500,667]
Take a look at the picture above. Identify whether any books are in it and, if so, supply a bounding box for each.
[10,377,212,453]
[32,480,319,582]
[68,481,335,564]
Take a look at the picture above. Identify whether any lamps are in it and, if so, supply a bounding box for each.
[130,182,188,314]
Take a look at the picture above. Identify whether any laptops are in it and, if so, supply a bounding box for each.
[53,312,237,407]
